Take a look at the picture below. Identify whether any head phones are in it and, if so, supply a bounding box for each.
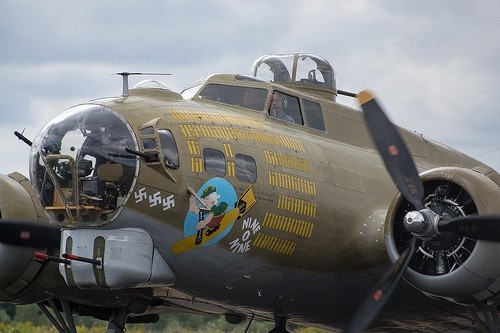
[283,94,287,110]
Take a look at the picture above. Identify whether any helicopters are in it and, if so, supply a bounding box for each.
[0,52,500,333]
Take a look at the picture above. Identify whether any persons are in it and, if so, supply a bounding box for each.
[269,92,294,124]
